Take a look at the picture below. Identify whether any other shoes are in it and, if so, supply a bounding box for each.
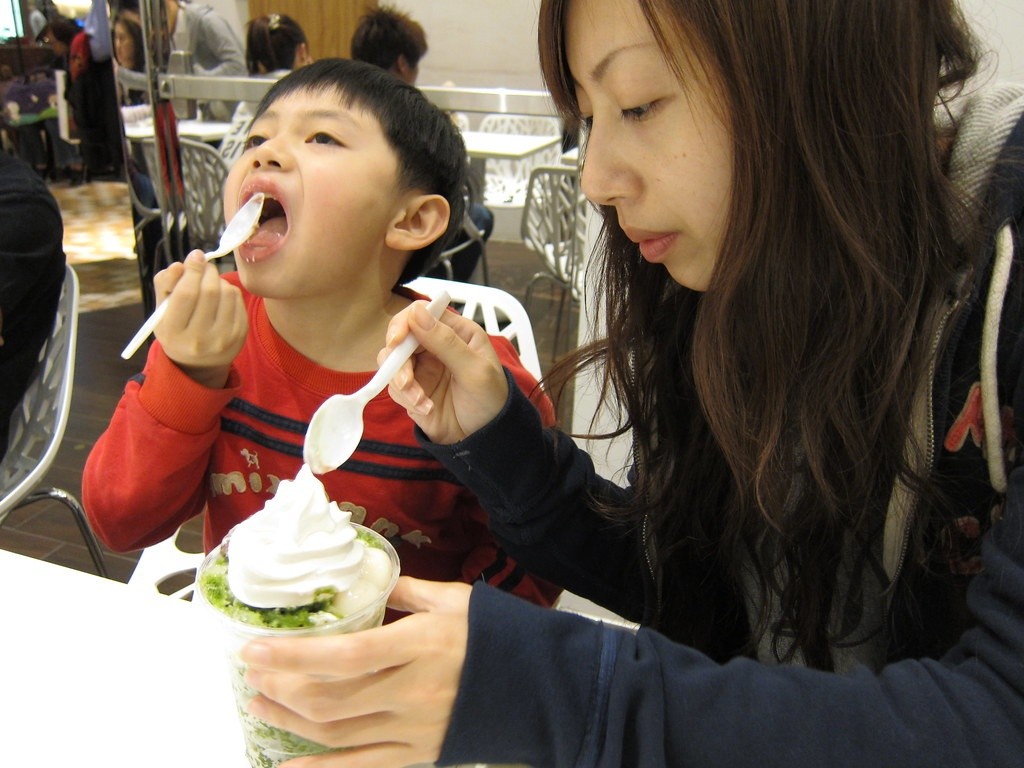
[60,165,84,181]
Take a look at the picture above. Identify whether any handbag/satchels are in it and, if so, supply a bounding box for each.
[2,64,59,127]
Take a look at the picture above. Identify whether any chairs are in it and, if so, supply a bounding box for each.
[423,129,591,302]
[140,137,240,276]
[0,263,112,581]
[124,272,547,603]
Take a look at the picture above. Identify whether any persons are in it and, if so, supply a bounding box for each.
[0,0,243,462]
[352,8,492,278]
[83,58,564,607]
[218,13,312,171]
[240,0,1024,768]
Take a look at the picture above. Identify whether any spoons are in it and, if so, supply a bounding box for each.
[121,192,266,361]
[303,288,451,475]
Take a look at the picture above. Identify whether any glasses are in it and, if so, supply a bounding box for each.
[37,36,50,48]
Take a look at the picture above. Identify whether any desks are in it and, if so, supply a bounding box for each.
[446,112,567,209]
[123,113,234,145]
[0,547,535,768]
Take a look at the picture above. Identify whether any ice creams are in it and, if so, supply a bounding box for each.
[198,464,393,768]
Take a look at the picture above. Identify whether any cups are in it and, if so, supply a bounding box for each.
[194,523,402,768]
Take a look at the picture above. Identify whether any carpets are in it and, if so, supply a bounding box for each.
[44,182,156,315]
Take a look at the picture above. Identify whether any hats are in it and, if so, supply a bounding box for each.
[29,9,49,42]
